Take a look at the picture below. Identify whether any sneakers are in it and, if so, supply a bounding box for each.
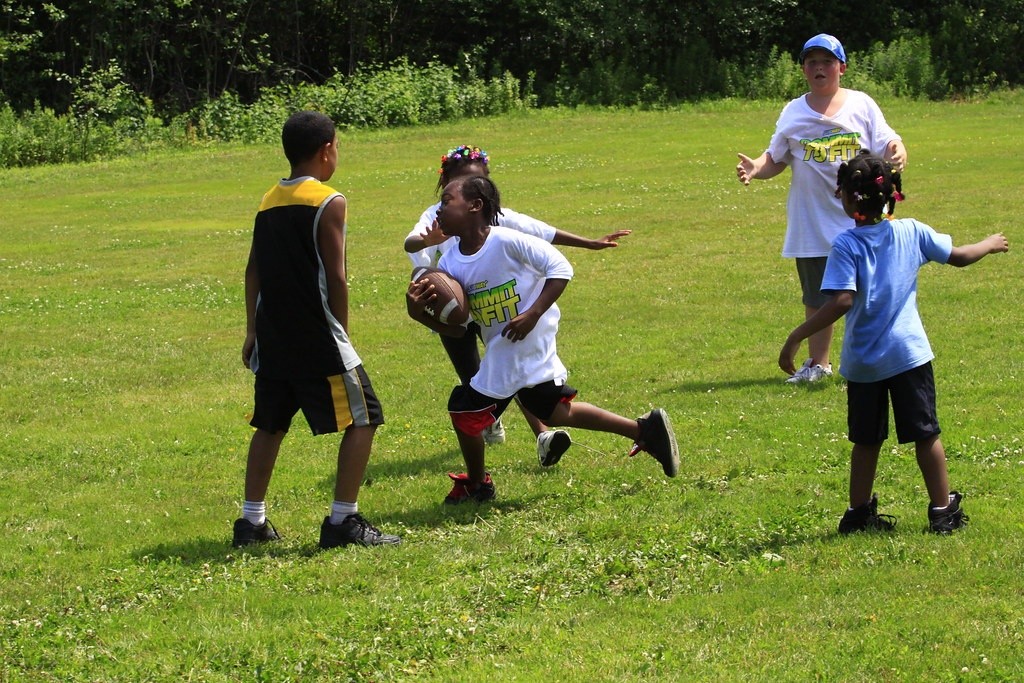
[536,430,572,466]
[628,409,679,477]
[783,357,833,382]
[837,492,896,533]
[483,421,506,445]
[926,489,969,533]
[229,515,279,545]
[444,472,495,502]
[319,514,402,546]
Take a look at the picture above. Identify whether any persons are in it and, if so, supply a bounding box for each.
[398,142,634,466]
[771,150,1011,539]
[401,174,682,509]
[228,111,405,548]
[736,31,916,385]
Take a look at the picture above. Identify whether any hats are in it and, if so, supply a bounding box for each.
[798,32,847,64]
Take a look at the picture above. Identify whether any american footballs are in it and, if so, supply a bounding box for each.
[411,267,469,324]
